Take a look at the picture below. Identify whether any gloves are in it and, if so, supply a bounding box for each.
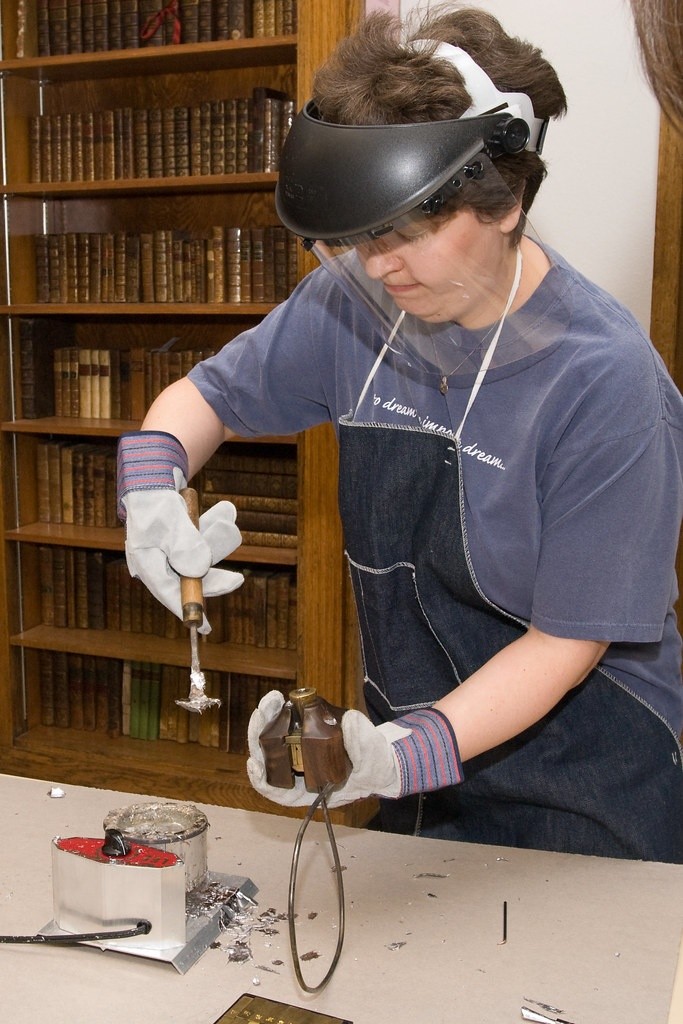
[116,430,245,635]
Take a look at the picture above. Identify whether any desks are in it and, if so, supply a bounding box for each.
[2,771,683,1024]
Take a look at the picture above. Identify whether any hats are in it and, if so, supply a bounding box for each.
[275,37,547,242]
[246,690,465,808]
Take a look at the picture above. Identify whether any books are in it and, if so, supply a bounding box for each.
[37,546,296,653]
[38,650,296,757]
[20,318,216,420]
[34,1,294,57]
[36,441,297,548]
[34,225,298,302]
[27,89,294,183]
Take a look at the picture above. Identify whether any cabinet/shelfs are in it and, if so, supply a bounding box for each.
[0,0,392,824]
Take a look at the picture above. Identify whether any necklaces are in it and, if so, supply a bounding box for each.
[420,239,527,396]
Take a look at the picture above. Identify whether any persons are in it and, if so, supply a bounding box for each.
[119,7,683,862]
